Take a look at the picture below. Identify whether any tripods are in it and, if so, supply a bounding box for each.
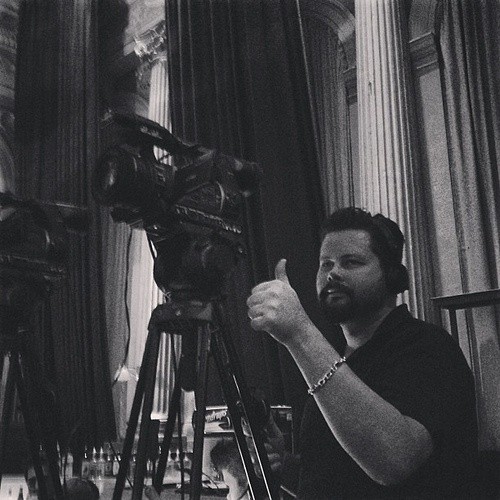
[0,253,67,499]
[108,241,280,500]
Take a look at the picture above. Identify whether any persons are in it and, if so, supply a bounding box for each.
[248,207,478,500]
[209,440,252,500]
[62,477,100,500]
[25,462,51,496]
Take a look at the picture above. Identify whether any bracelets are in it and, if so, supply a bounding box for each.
[308,356,347,395]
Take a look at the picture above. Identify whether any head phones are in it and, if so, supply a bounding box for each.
[368,214,410,295]
